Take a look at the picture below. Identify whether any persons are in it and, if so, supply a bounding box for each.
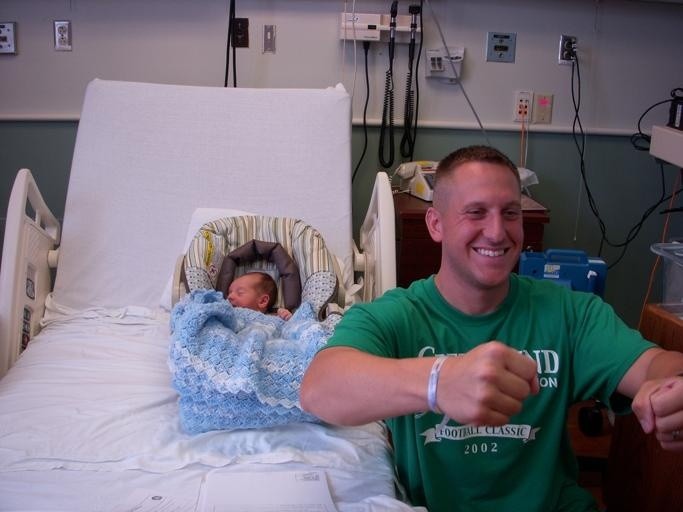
[300,145,682,512]
[224,271,293,320]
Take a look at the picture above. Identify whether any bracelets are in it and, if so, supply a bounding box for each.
[426,355,449,413]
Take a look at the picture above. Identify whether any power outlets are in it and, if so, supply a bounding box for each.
[54,20,71,49]
[561,33,580,66]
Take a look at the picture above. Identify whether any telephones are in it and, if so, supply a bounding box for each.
[396,161,441,202]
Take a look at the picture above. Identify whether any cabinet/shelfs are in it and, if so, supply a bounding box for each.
[388,178,550,290]
[641,303,682,512]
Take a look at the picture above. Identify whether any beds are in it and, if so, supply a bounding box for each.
[1,79,400,510]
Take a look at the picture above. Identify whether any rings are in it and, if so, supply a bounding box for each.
[671,429,681,440]
